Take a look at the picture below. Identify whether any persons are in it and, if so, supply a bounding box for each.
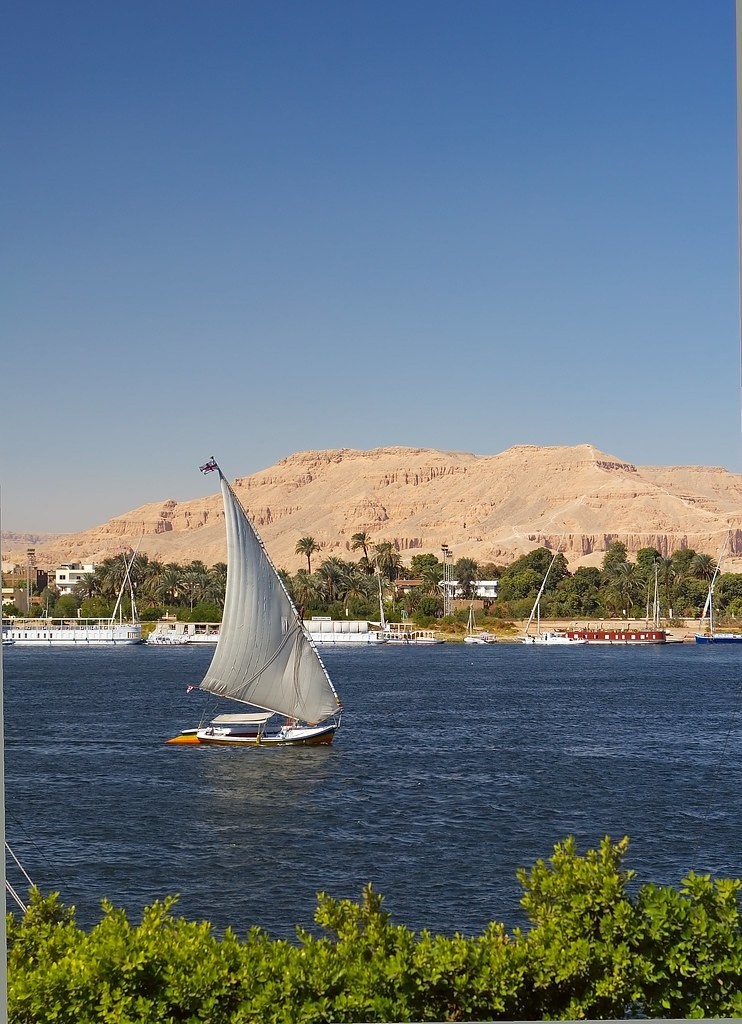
[206,723,212,735]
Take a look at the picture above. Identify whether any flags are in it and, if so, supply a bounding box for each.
[186,686,193,693]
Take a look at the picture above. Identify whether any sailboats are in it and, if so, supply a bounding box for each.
[164,456,344,747]
[464,604,497,645]
[695,587,742,644]
[376,567,446,646]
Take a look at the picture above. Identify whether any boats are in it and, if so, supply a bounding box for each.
[522,604,588,645]
[146,630,188,645]
[146,620,386,645]
[2,639,15,645]
[2,618,142,645]
